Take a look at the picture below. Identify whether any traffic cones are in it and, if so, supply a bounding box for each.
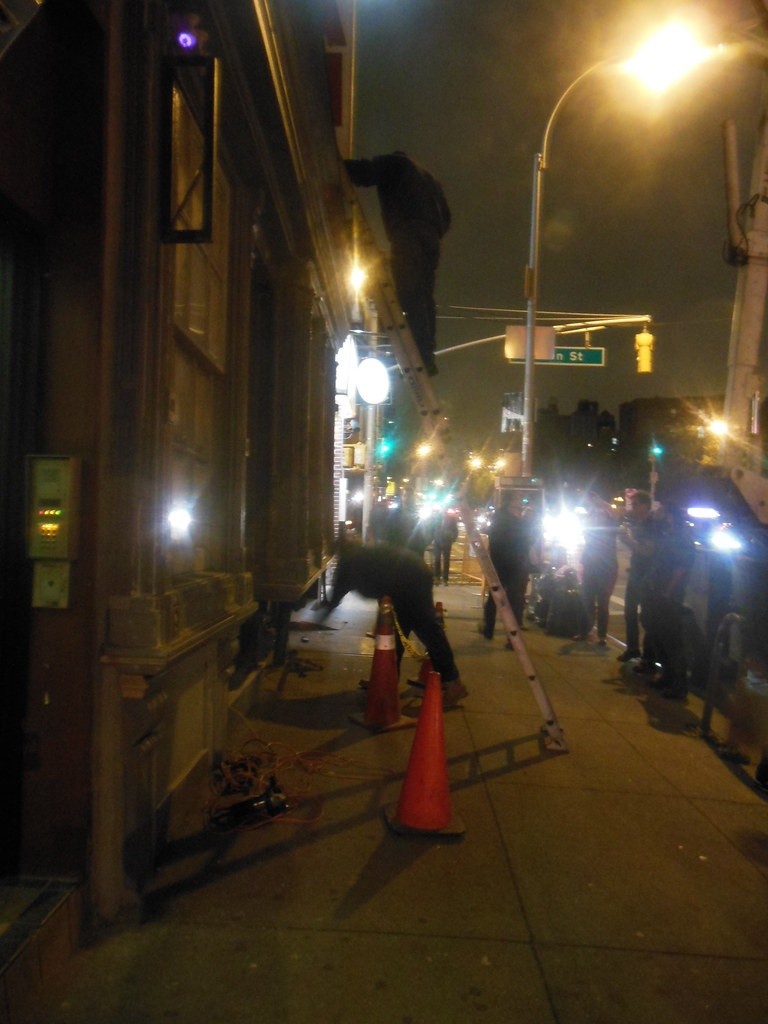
[404,600,455,691]
[382,671,465,837]
[346,594,418,734]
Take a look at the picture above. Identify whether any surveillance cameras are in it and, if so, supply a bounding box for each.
[177,29,208,49]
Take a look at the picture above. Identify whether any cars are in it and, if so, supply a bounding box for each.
[373,486,768,583]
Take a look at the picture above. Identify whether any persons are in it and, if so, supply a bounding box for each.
[330,535,469,709]
[371,501,458,587]
[612,484,742,705]
[474,494,623,651]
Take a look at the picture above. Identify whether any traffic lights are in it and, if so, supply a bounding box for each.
[651,441,662,461]
[378,439,391,463]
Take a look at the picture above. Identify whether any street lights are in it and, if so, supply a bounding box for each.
[520,22,712,488]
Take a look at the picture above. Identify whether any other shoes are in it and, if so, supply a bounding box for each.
[426,364,439,377]
[479,624,494,639]
[442,679,468,706]
[635,659,656,673]
[618,649,640,662]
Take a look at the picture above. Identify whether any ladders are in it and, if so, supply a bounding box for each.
[343,165,569,752]
